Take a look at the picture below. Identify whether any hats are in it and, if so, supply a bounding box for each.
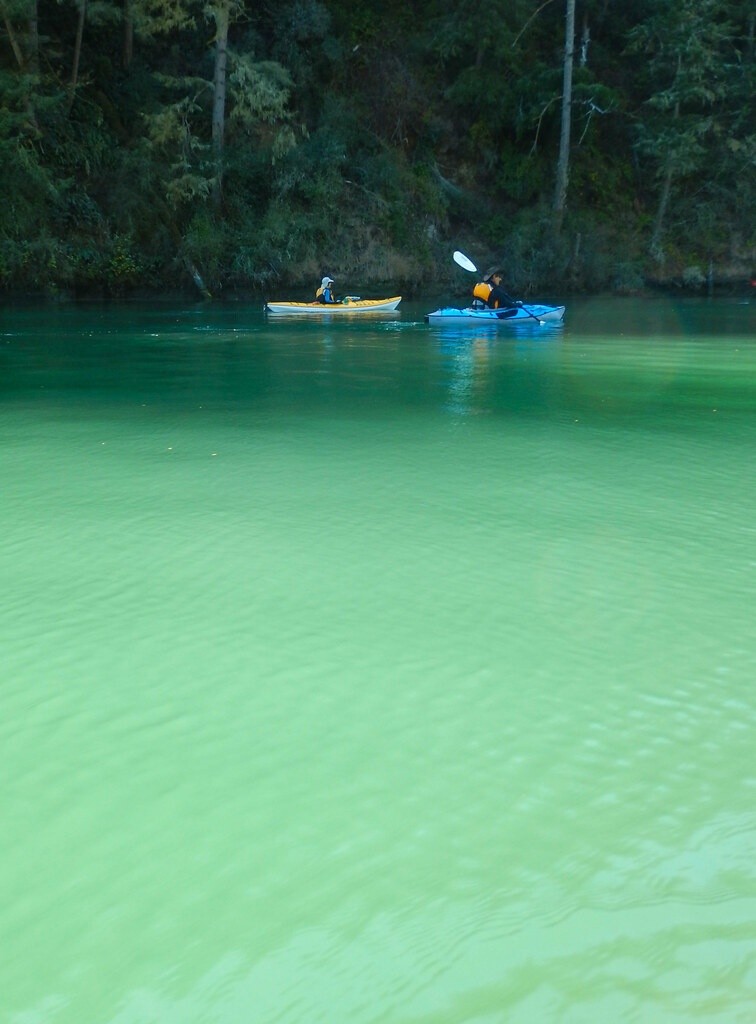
[483,266,505,282]
[320,277,333,288]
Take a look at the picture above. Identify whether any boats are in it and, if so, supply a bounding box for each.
[423,296,566,327]
[263,295,402,313]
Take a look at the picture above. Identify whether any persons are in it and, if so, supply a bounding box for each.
[472,266,522,310]
[320,277,343,304]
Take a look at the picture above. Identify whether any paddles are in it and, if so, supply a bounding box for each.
[453,250,546,324]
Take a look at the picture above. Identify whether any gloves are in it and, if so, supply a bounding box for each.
[516,303,522,307]
